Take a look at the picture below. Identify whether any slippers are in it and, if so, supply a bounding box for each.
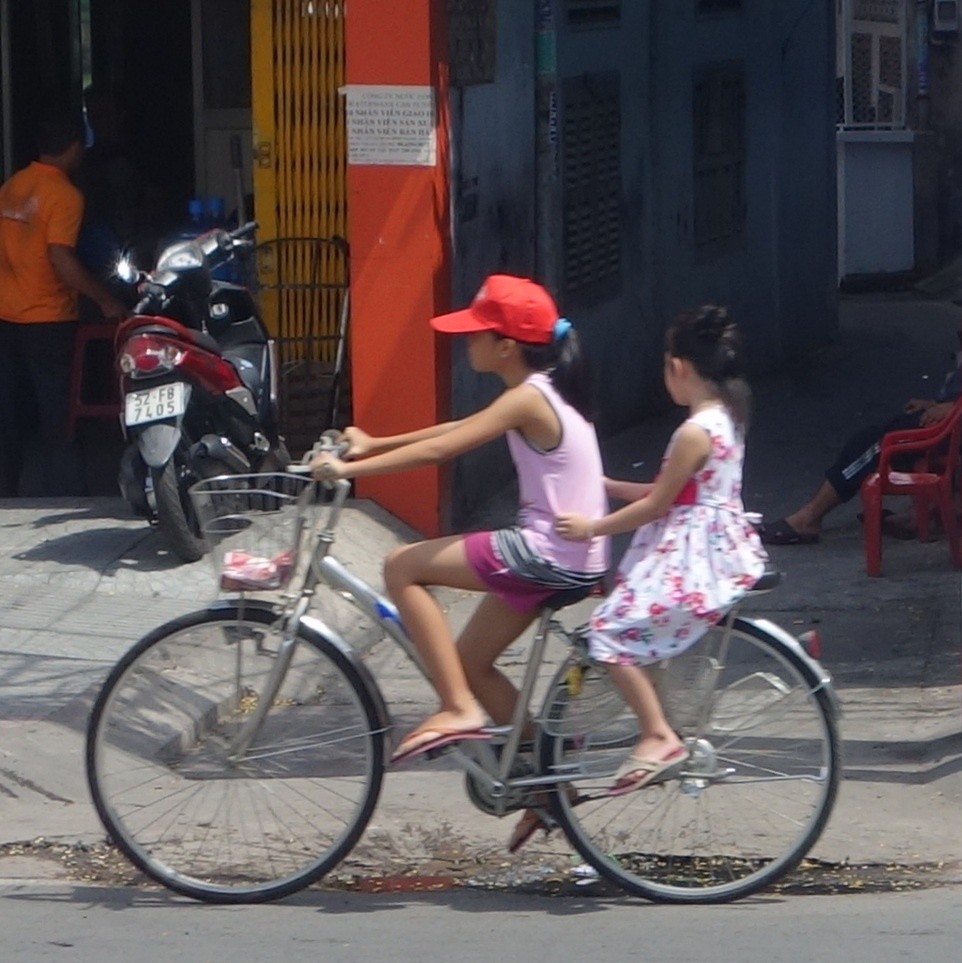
[858,508,917,539]
[758,519,818,543]
[390,727,493,763]
[509,794,590,852]
[606,748,690,796]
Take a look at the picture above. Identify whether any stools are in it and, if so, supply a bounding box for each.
[65,323,121,438]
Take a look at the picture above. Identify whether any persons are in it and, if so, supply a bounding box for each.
[307,275,610,856]
[755,330,962,544]
[0,97,129,497]
[555,305,766,796]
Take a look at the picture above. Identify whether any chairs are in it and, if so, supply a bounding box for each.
[859,390,962,578]
[916,455,962,541]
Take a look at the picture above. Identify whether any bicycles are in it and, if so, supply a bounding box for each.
[84,429,853,905]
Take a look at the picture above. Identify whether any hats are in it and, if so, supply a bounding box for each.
[429,275,557,342]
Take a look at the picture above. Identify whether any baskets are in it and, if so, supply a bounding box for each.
[189,473,317,591]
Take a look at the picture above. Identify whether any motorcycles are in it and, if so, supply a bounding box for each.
[117,213,298,562]
